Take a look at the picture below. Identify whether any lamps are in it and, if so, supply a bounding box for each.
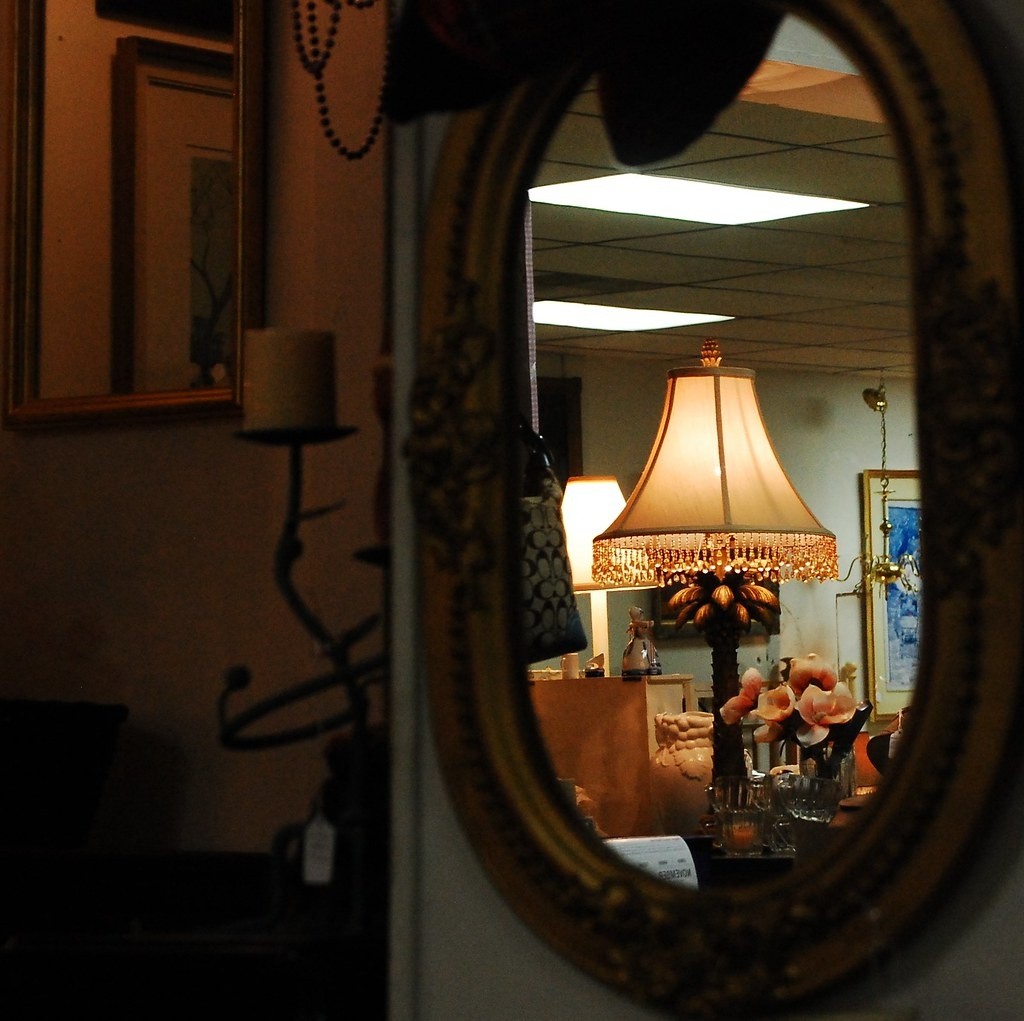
[591,338,838,845]
[560,474,660,676]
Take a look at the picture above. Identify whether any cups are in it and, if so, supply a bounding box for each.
[560,653,580,679]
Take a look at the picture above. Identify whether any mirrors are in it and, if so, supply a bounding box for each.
[403,0,1023,1021]
[0,0,258,432]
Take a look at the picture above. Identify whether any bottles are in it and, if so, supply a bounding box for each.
[706,773,843,860]
[648,710,753,836]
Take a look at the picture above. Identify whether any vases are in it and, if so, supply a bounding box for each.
[648,711,755,837]
[800,745,857,797]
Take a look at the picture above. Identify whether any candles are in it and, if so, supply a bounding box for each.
[240,327,337,429]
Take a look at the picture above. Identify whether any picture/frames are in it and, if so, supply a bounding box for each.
[860,469,926,724]
[114,33,241,393]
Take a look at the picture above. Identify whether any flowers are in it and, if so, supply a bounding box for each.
[719,652,869,774]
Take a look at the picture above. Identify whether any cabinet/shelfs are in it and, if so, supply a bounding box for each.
[531,673,698,839]
